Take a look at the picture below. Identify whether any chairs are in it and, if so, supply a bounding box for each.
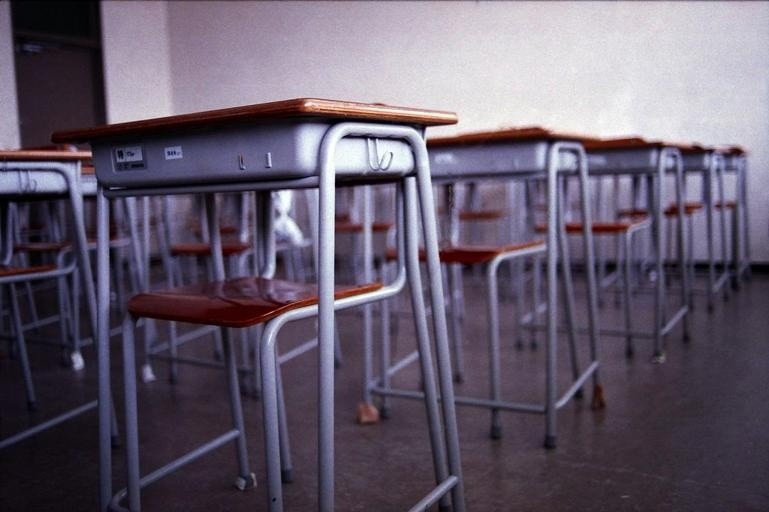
[124,174,452,509]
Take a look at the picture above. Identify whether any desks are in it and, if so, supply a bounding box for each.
[52,98,469,509]
[2,125,753,457]
